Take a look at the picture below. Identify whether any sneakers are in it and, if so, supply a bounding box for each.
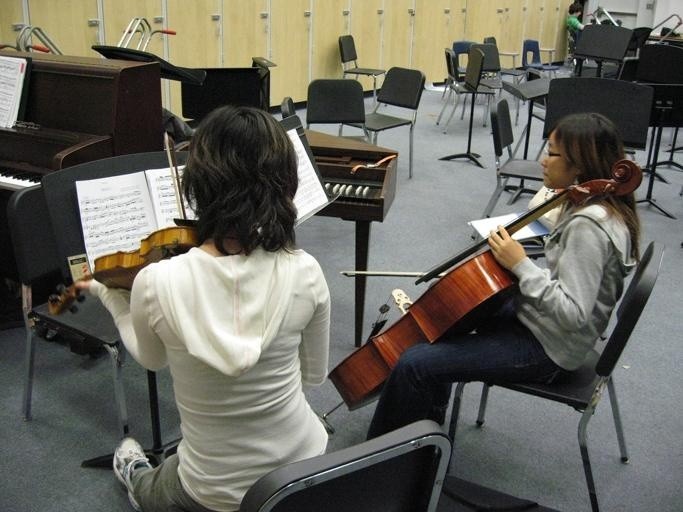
[111,434,155,512]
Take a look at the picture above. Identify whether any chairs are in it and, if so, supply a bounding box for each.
[8,182,131,438]
[339,66,425,178]
[482,98,546,220]
[446,235,671,512]
[437,19,639,206]
[306,78,370,143]
[339,35,389,112]
[230,419,450,512]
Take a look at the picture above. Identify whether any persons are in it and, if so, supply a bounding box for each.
[361,111,642,443]
[566,3,592,66]
[74,103,331,510]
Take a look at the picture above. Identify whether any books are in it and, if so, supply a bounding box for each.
[0,55,32,131]
[73,164,200,278]
[282,127,329,223]
[465,183,563,257]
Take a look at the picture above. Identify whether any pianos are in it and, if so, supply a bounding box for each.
[303,130,400,223]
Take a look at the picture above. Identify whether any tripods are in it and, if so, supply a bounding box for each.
[649,125,683,171]
[503,99,539,205]
[633,107,676,218]
[81,370,183,473]
[438,94,485,168]
[639,125,671,184]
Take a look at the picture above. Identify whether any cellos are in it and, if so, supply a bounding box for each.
[328,160,642,410]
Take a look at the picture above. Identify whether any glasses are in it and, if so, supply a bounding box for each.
[543,146,561,159]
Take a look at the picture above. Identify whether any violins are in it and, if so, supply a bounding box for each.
[47,227,201,316]
